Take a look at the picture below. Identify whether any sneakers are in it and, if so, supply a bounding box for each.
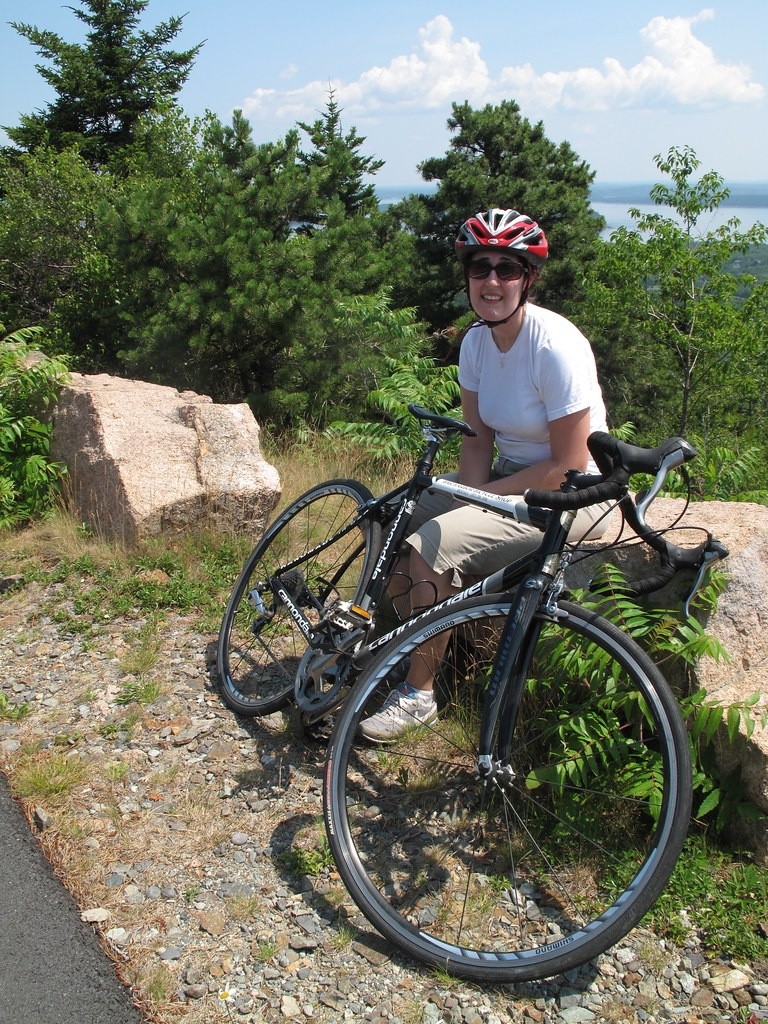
[322,655,411,688]
[357,689,440,745]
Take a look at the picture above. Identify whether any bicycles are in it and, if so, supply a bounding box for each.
[210,399,732,984]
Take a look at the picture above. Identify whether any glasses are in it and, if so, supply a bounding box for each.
[462,259,529,281]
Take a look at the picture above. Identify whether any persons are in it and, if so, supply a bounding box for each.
[318,208,616,743]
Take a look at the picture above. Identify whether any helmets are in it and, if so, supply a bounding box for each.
[455,207,549,274]
